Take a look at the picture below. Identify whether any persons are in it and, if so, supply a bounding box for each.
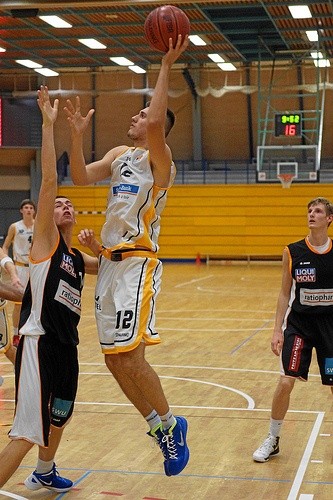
[63,32,205,477]
[253,198,333,463]
[0,84,100,494]
[0,198,36,388]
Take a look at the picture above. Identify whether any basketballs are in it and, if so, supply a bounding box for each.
[144,5,190,53]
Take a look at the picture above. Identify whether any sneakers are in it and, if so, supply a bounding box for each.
[13,334,20,347]
[160,415,190,475]
[23,462,73,493]
[252,433,279,462]
[146,423,172,476]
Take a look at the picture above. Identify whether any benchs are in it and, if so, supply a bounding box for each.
[52,170,333,259]
